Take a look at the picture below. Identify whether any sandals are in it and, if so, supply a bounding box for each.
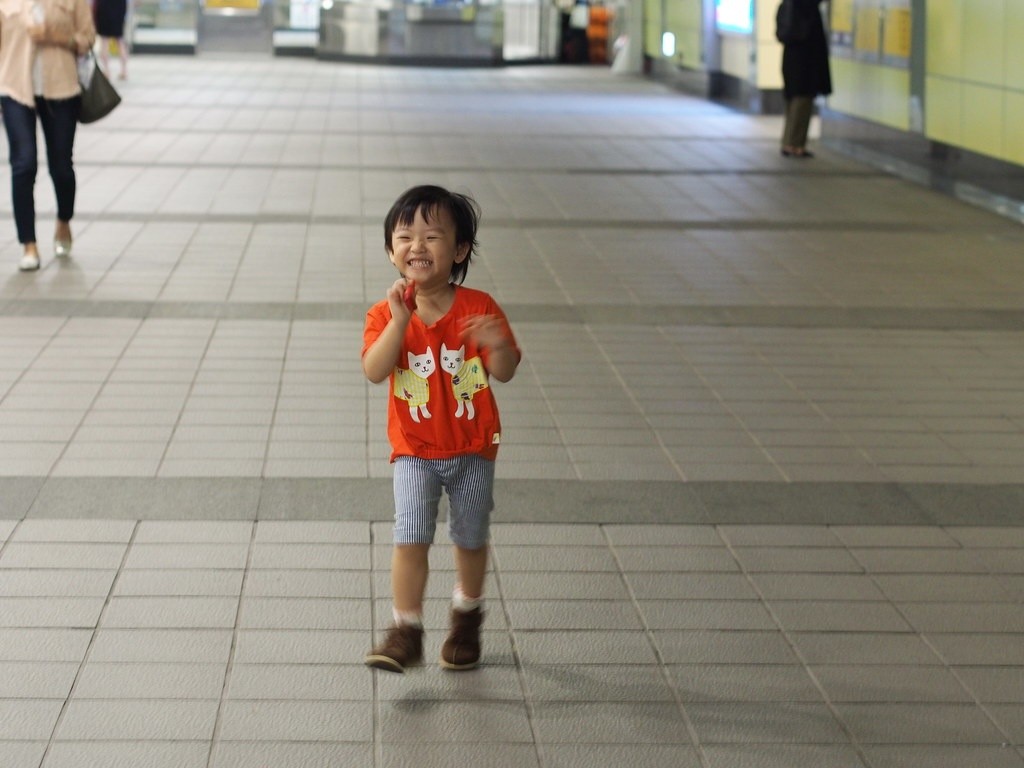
[53,234,72,256]
[19,253,40,270]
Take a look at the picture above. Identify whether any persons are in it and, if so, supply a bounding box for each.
[1,1,99,271]
[776,0,835,158]
[94,1,130,83]
[355,184,525,672]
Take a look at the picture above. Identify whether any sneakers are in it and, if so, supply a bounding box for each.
[440,607,486,669]
[364,626,426,673]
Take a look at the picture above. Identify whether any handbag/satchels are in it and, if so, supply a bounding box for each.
[77,51,123,125]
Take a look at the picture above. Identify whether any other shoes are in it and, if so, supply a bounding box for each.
[781,148,815,158]
